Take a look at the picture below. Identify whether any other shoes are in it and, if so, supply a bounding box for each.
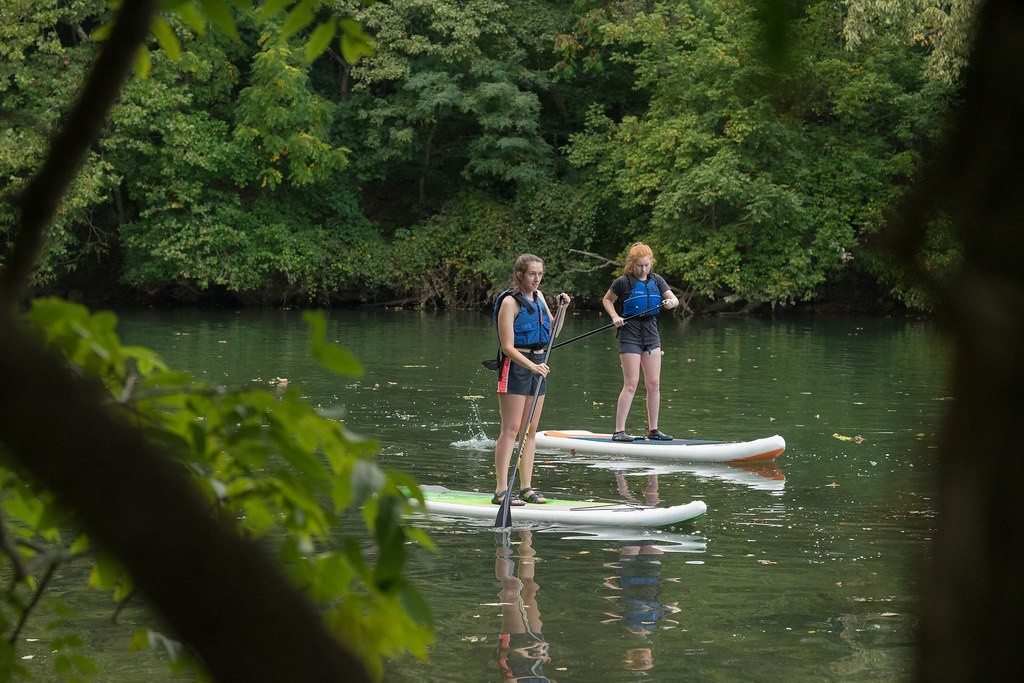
[648,431,674,440]
[612,431,634,442]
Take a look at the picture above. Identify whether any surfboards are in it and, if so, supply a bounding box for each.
[533,431,785,461]
[397,484,710,527]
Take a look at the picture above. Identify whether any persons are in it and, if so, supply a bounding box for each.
[492,252,571,505]
[606,241,680,441]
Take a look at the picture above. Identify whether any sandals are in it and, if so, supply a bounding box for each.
[519,487,546,503]
[491,491,527,506]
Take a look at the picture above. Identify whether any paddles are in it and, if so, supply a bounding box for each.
[495,293,567,529]
[481,299,669,371]
